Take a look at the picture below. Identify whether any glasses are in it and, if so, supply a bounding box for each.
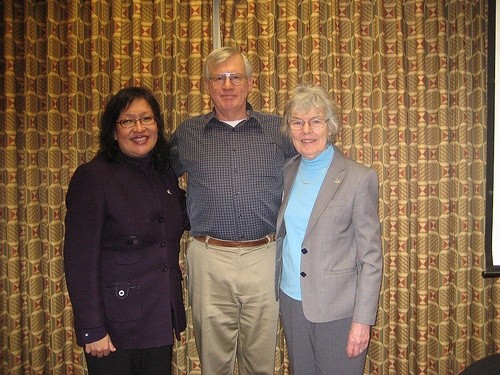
[209,73,247,86]
[115,116,157,128]
[287,118,329,130]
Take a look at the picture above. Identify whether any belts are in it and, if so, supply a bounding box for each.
[194,232,275,247]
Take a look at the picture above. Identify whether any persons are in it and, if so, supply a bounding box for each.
[275,84,384,374]
[63,87,191,375]
[168,47,300,375]
